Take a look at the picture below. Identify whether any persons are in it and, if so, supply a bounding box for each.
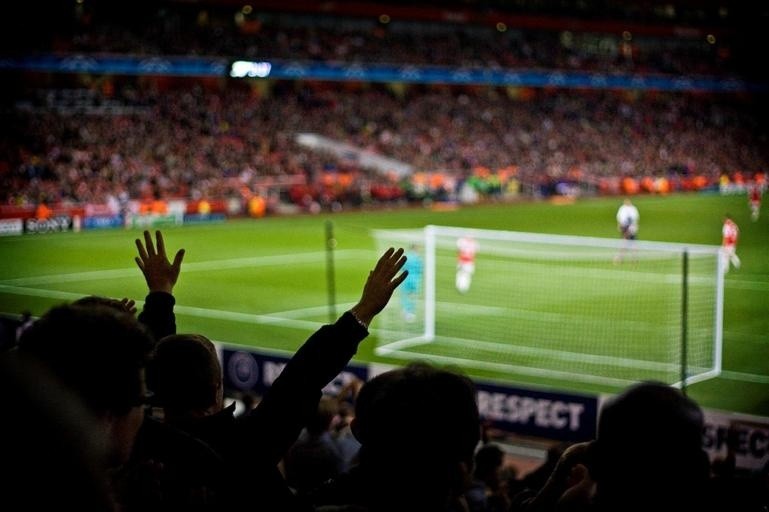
[130,227,411,510]
[717,211,745,276]
[398,242,422,321]
[1,297,767,512]
[612,194,641,267]
[1,1,769,224]
[450,230,479,293]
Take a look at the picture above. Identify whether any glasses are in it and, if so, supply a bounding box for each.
[135,391,154,409]
